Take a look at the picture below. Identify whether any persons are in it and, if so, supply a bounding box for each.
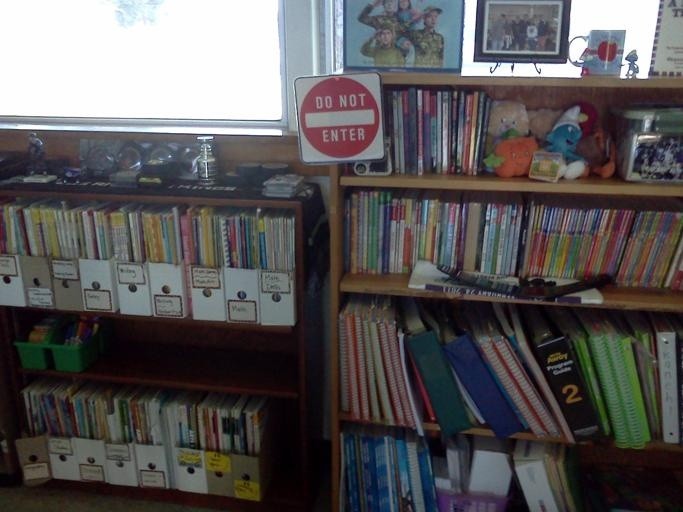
[359,24,405,66]
[357,0,401,49]
[491,13,548,51]
[632,140,682,181]
[399,6,443,67]
[380,1,421,54]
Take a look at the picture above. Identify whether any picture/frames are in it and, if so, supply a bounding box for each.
[473,0,572,65]
[343,0,464,73]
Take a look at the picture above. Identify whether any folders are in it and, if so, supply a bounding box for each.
[400,299,525,440]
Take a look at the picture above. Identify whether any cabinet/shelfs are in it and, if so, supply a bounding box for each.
[1,163,323,510]
[329,73,683,510]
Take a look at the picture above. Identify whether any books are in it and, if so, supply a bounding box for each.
[343,86,486,176]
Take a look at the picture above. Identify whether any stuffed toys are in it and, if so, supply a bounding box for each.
[482,99,619,181]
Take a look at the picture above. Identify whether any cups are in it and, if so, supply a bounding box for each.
[566,29,627,79]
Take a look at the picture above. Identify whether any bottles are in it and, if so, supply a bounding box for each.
[194,135,217,188]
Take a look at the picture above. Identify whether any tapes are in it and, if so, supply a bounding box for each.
[236,163,288,175]
